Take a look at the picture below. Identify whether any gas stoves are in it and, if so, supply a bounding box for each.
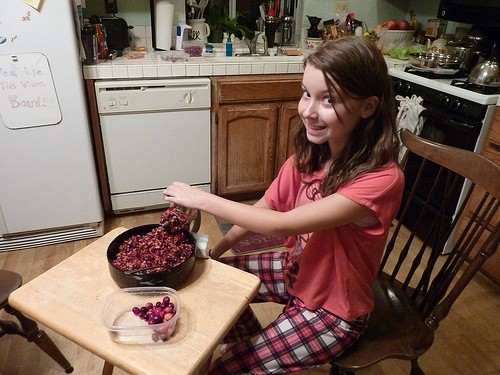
[406,65,500,96]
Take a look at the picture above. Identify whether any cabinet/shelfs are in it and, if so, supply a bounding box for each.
[452,108,500,294]
[209,75,306,200]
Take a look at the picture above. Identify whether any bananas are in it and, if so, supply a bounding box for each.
[409,16,419,29]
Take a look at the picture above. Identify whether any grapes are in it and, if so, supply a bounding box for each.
[133,296,177,342]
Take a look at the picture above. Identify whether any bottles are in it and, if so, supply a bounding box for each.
[226,38,232,56]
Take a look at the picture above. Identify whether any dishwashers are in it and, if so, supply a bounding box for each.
[95,80,212,214]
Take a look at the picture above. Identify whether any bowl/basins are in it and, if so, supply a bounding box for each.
[373,29,417,51]
[107,224,195,294]
[102,286,181,343]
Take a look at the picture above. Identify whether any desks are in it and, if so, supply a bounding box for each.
[8,226,261,375]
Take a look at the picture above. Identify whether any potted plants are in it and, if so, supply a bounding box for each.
[210,6,252,57]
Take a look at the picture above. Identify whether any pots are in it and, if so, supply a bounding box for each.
[468,58,500,86]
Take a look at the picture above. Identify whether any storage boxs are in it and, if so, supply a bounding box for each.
[426,18,448,37]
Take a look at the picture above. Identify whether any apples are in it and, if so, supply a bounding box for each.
[384,19,409,30]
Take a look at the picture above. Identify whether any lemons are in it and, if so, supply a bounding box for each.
[390,46,427,60]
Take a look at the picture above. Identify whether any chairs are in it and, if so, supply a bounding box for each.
[323,130,500,375]
[0,269,74,374]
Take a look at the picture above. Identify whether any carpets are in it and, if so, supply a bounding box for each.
[215,202,285,256]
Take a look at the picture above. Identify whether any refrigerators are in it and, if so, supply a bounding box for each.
[0,1,103,237]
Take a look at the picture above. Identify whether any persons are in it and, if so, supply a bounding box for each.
[164,36,407,375]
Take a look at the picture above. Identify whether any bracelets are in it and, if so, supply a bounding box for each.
[209,249,212,257]
